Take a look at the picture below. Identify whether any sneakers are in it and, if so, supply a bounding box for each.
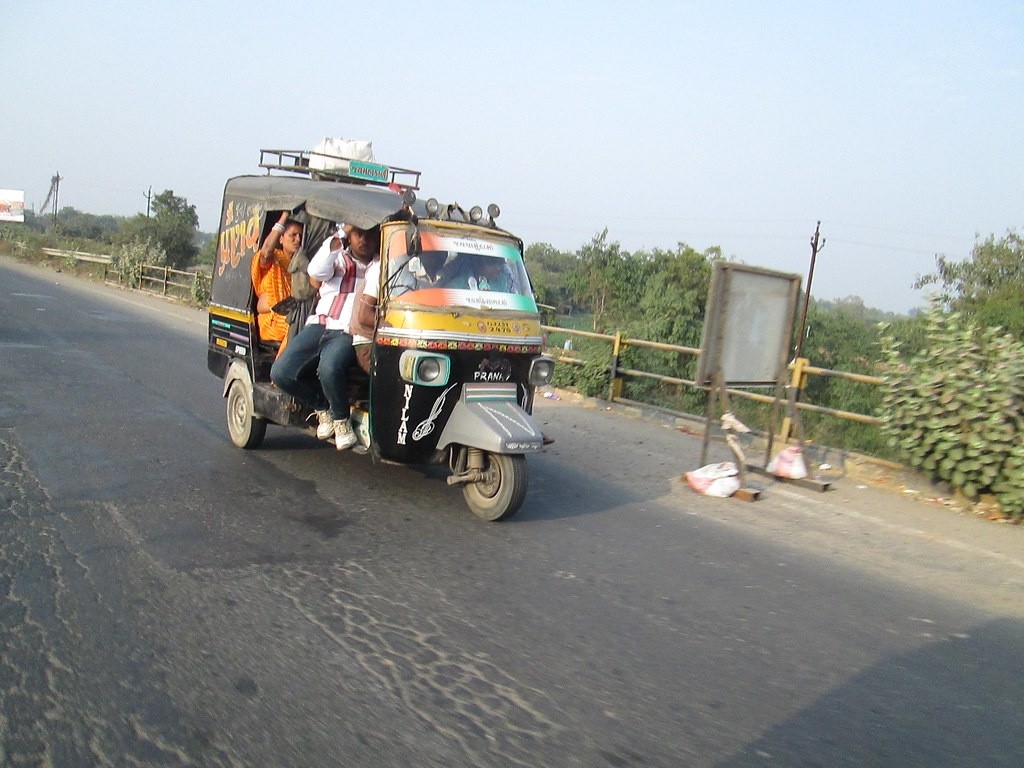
[333,416,357,451]
[306,409,334,440]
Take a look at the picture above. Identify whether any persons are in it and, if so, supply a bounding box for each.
[248,212,305,342]
[269,222,380,450]
[349,251,448,377]
[441,256,507,293]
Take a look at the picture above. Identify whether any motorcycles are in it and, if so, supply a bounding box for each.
[206,149,556,522]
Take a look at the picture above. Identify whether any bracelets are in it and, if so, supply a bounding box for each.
[273,223,286,234]
[338,229,348,240]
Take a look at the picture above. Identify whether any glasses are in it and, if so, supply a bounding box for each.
[487,256,506,266]
